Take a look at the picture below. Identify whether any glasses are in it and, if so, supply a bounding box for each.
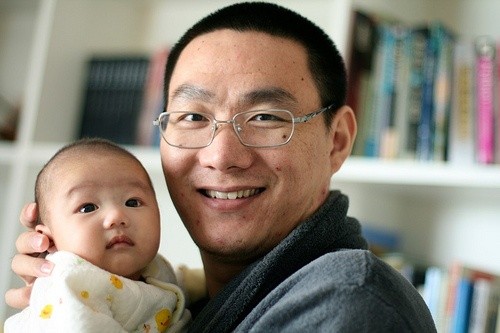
[153,104,333,149]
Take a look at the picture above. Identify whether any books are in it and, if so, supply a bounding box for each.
[72,12,500,333]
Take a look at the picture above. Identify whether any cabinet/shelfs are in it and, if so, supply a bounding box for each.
[0,0,500,333]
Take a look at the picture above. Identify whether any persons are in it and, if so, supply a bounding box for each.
[4,139,191,333]
[4,0,437,333]
